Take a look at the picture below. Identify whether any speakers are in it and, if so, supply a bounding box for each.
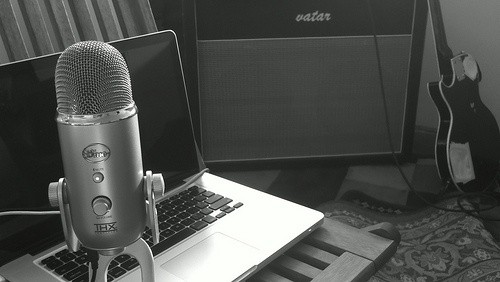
[181,0,427,171]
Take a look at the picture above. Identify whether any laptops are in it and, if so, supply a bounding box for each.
[0,30,325,282]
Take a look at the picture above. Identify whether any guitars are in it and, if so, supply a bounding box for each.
[426,1,500,195]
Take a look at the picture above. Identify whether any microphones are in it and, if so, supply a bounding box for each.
[54,40,165,282]
